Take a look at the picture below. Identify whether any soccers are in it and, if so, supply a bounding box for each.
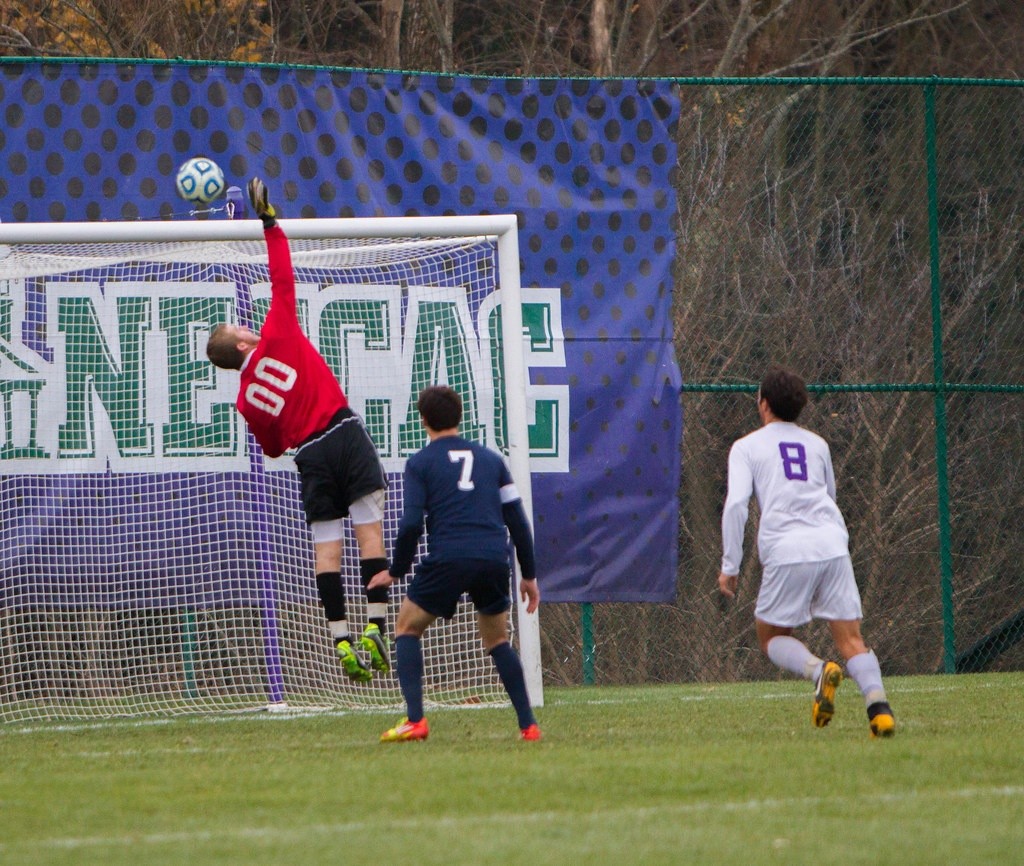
[176,156,225,205]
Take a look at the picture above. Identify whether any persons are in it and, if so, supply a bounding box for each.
[205,177,542,743]
[718,369,896,740]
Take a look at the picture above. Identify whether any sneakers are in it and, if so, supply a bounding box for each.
[521,724,540,740]
[336,640,372,682]
[360,623,390,672]
[813,660,842,728]
[869,713,893,738]
[380,717,428,742]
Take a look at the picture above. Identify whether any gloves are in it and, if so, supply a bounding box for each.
[245,178,276,228]
